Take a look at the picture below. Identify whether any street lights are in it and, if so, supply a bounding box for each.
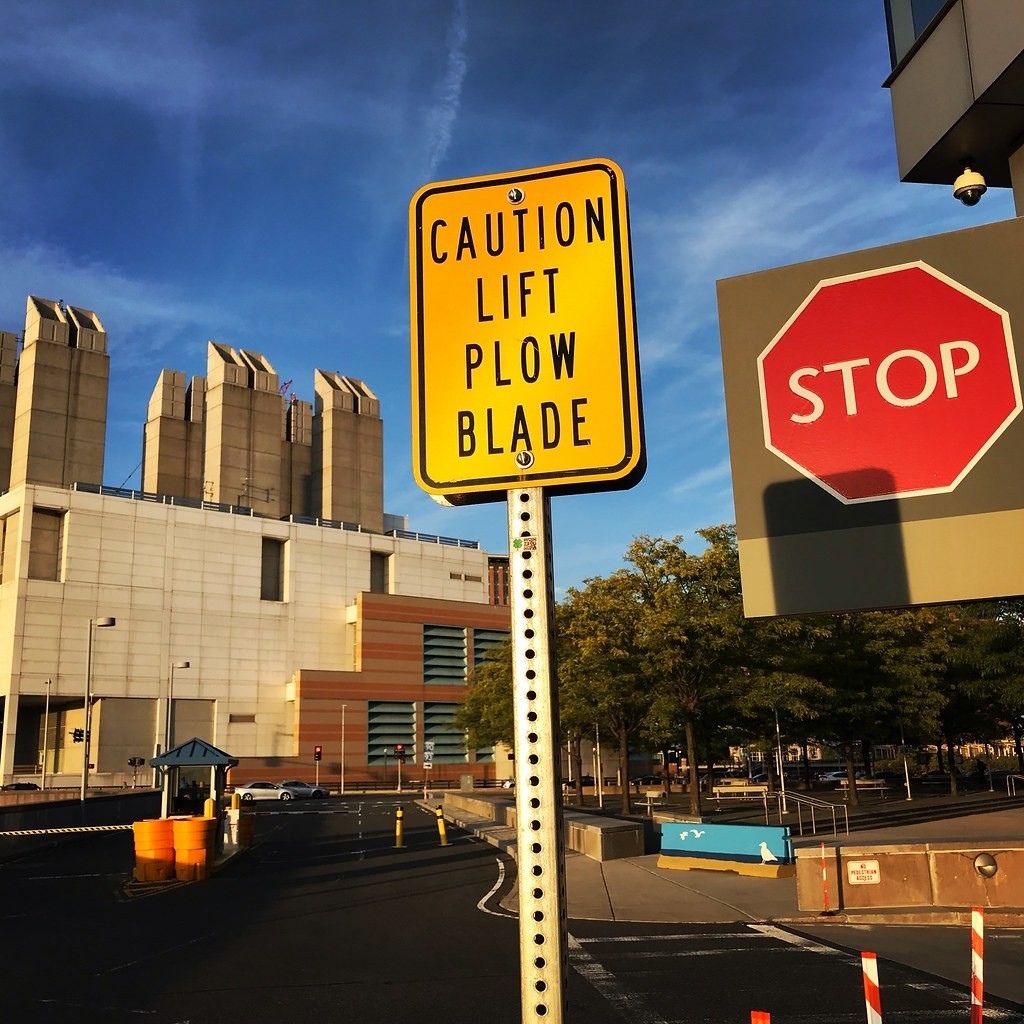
[40,677,54,792]
[80,614,118,802]
[340,704,348,794]
[165,660,190,753]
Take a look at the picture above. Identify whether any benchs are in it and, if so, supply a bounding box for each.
[706,777,775,812]
[633,790,680,816]
[836,780,892,799]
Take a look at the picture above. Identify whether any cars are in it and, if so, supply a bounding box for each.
[277,779,331,799]
[703,772,742,781]
[922,771,960,782]
[753,773,789,783]
[563,776,606,788]
[818,770,851,782]
[871,771,905,781]
[965,771,986,776]
[234,780,296,802]
[630,775,672,785]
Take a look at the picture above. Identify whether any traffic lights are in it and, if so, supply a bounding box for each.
[393,744,406,766]
[312,745,323,762]
[88,763,93,768]
[127,757,145,766]
[74,729,90,745]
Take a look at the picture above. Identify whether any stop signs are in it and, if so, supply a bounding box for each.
[753,255,1024,510]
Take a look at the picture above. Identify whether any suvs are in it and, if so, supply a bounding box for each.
[3,783,40,790]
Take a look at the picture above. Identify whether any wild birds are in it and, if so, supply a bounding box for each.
[690,830,705,838]
[680,831,688,840]
[758,842,778,864]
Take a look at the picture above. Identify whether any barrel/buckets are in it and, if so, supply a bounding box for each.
[173,818,217,881]
[134,819,174,881]
